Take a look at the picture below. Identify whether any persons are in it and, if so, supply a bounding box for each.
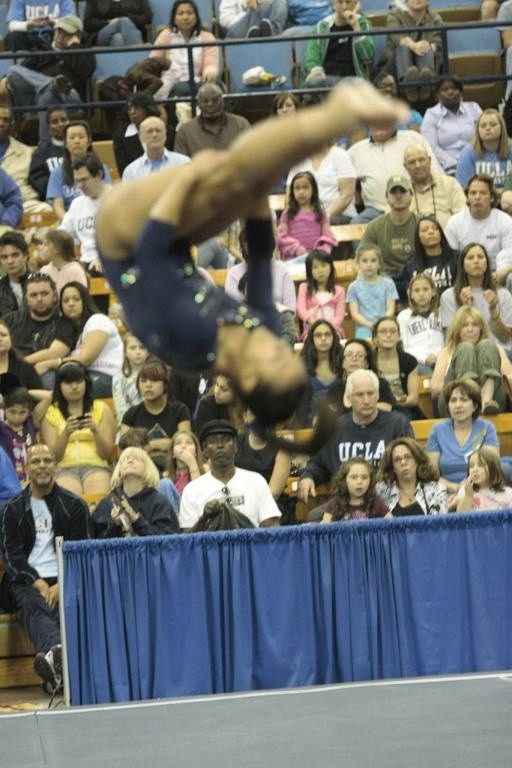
[1,444,92,697]
[2,1,511,538]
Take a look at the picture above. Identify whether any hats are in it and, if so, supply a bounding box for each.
[387,174,411,194]
[53,15,86,35]
[199,419,239,445]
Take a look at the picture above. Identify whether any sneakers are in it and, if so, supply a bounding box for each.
[44,644,63,689]
[260,17,274,36]
[33,652,63,696]
[403,66,421,103]
[246,25,261,38]
[420,67,433,101]
[482,398,500,415]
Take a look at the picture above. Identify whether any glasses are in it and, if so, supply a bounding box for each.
[313,332,333,338]
[26,272,51,281]
[341,351,368,361]
